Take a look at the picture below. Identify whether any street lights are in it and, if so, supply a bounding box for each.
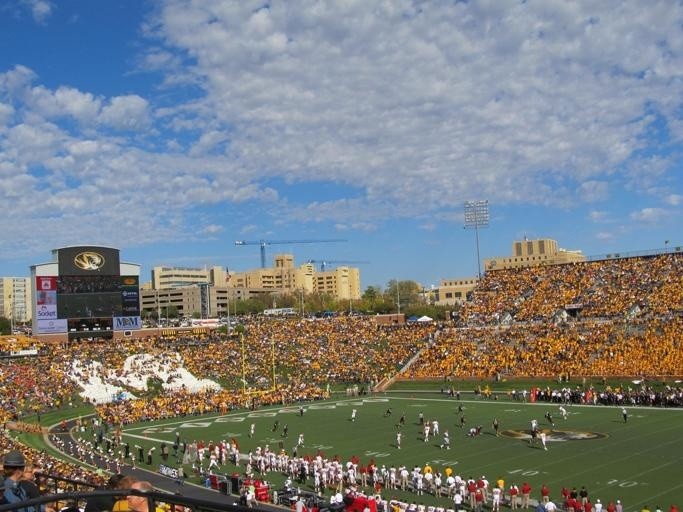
[463,200,489,281]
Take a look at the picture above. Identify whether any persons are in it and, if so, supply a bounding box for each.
[0,252,682,511]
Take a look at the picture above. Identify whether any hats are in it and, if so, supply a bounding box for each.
[3,451,26,466]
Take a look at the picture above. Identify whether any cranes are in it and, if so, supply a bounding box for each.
[233,238,348,268]
[307,259,369,271]
